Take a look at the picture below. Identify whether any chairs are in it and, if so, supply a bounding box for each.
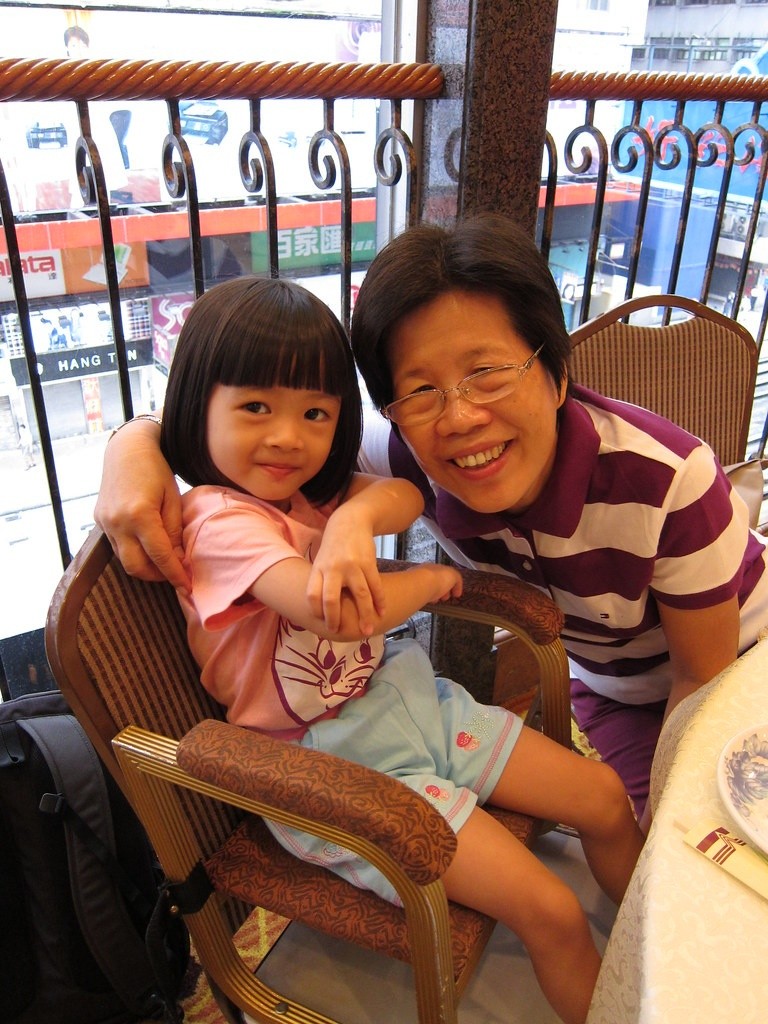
[46,523,571,1024]
[429,293,755,730]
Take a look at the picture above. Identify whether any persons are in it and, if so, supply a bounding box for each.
[16,425,36,469]
[160,275,646,1024]
[561,283,575,301]
[92,212,768,839]
[750,283,759,310]
[722,291,735,319]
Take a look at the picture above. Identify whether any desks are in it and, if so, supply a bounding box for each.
[581,635,768,1024]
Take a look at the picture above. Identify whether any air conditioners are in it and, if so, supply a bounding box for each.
[718,213,752,238]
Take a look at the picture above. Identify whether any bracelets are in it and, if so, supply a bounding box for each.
[102,413,162,442]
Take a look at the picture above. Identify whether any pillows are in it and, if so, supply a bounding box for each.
[722,458,765,528]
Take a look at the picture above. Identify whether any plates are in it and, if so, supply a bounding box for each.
[717,723,767,855]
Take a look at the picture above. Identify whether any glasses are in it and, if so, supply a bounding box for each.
[379,345,549,428]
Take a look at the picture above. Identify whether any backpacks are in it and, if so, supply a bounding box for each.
[0,688,191,1024]
